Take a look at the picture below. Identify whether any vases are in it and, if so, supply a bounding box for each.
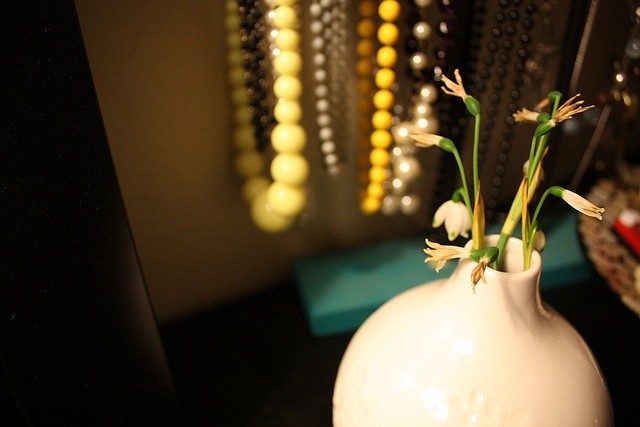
[329,231,619,427]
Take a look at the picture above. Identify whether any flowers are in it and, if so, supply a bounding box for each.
[409,67,606,295]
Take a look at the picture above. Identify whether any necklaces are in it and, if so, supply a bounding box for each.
[237,0,278,153]
[382,0,536,230]
[224,0,312,234]
[309,0,351,179]
[355,0,402,216]
